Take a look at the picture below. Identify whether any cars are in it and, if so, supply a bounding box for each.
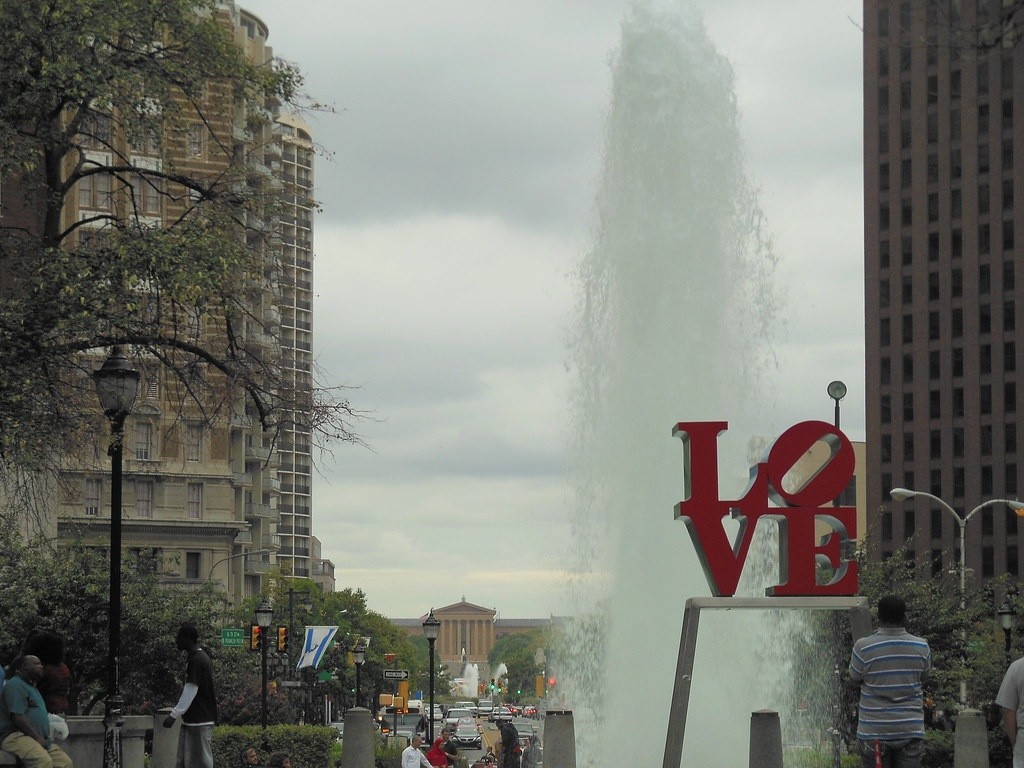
[328,722,344,738]
[381,713,429,747]
[424,701,543,768]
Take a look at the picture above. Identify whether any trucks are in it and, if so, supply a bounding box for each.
[386,700,424,714]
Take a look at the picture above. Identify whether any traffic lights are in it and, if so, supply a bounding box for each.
[250,625,261,651]
[277,625,289,653]
[491,679,495,690]
[498,682,502,693]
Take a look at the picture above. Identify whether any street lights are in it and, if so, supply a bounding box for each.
[423,609,441,748]
[254,600,274,750]
[998,602,1017,672]
[352,644,365,707]
[890,489,1024,705]
[91,345,142,768]
[208,548,271,614]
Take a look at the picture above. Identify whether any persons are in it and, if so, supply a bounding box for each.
[401,718,521,768]
[240,748,291,768]
[848,594,930,767]
[995,657,1024,768]
[0,654,74,768]
[162,624,219,768]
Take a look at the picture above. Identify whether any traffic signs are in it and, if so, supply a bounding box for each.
[384,669,408,680]
